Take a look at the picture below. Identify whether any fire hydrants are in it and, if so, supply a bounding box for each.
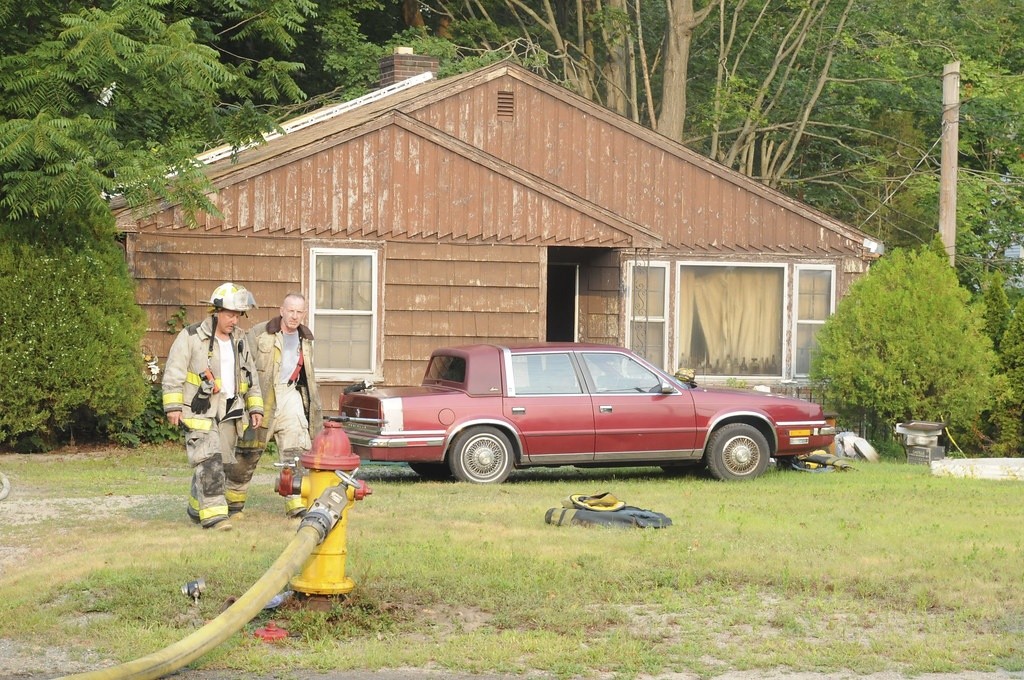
[271,413,377,606]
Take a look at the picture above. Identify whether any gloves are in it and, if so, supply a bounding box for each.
[191,380,214,415]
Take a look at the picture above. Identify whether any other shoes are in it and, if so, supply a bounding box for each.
[203,520,232,531]
[291,510,308,518]
[187,513,201,525]
[229,510,248,521]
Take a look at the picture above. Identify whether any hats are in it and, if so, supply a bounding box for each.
[207,304,248,318]
[570,492,626,512]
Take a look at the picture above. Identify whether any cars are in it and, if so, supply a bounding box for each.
[336,341,838,487]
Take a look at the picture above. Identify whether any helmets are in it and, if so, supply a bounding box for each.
[209,283,258,311]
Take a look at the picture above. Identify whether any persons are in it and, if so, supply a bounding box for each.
[162,282,263,533]
[226,291,320,522]
